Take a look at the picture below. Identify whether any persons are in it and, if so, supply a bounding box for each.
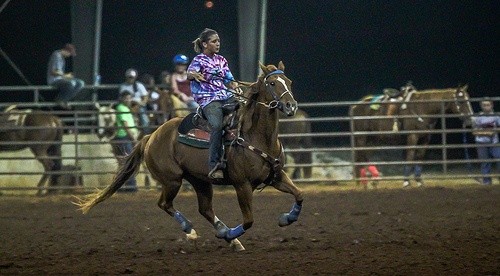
[471,97,500,184]
[48,44,86,110]
[187,29,243,178]
[112,91,137,192]
[117,54,194,127]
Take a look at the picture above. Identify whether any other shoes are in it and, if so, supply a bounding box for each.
[208,170,224,179]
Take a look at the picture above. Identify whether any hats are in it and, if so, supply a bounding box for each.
[173,54,189,65]
[64,43,76,56]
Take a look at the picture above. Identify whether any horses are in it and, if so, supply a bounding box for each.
[96,88,313,191]
[70,60,305,253]
[350,84,476,187]
[0,106,64,196]
